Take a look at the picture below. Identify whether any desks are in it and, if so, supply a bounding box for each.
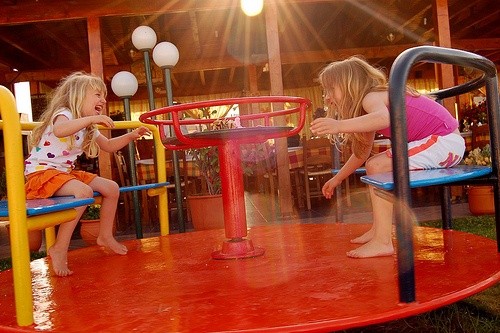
[459,132,491,152]
[272,139,392,173]
[136,158,205,233]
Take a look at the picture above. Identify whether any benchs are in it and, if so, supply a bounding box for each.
[331,165,493,190]
[0,181,170,216]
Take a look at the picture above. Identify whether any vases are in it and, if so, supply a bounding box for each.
[187,193,225,230]
[467,186,495,216]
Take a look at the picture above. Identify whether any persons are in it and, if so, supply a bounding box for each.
[310,57,466,258]
[23,73,152,277]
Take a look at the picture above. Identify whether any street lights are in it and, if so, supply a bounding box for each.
[131,25,158,182]
[111,70,143,238]
[152,41,186,233]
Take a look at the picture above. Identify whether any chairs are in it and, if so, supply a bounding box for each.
[114,150,150,227]
[469,123,491,151]
[262,135,351,212]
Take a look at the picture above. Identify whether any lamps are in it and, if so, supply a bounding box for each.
[10,63,25,72]
[110,25,185,239]
[385,27,401,43]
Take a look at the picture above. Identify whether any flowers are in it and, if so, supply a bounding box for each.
[464,144,491,166]
[168,96,242,196]
